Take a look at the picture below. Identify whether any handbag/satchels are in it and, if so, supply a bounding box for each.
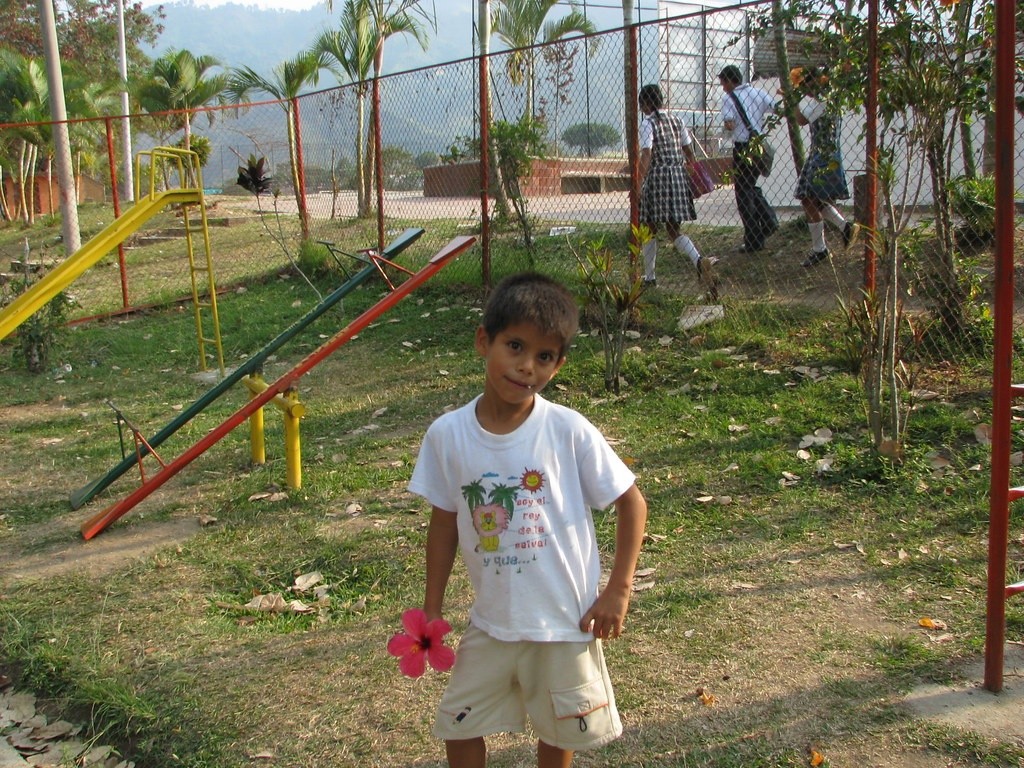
[747,129,772,176]
[685,162,715,198]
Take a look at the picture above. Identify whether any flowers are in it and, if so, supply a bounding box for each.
[387,609,455,678]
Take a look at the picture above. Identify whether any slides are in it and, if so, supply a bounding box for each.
[1,186,203,341]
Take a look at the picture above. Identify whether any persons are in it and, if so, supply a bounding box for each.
[795,69,860,267]
[407,272,647,768]
[638,85,720,288]
[718,65,785,253]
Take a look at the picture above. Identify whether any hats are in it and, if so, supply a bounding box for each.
[788,65,828,87]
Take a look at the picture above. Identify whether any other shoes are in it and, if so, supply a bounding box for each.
[740,241,764,252]
[800,248,829,267]
[638,275,657,287]
[697,256,719,287]
[842,222,859,251]
